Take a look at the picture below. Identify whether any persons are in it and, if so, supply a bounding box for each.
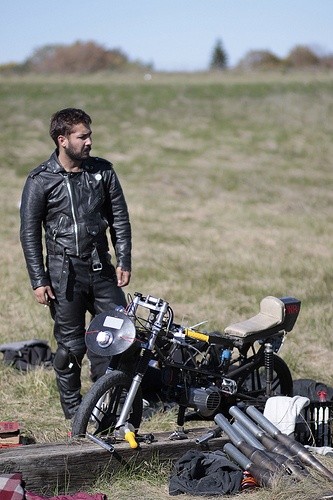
[18,107,135,422]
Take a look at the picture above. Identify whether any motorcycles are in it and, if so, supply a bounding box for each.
[69,288,304,443]
